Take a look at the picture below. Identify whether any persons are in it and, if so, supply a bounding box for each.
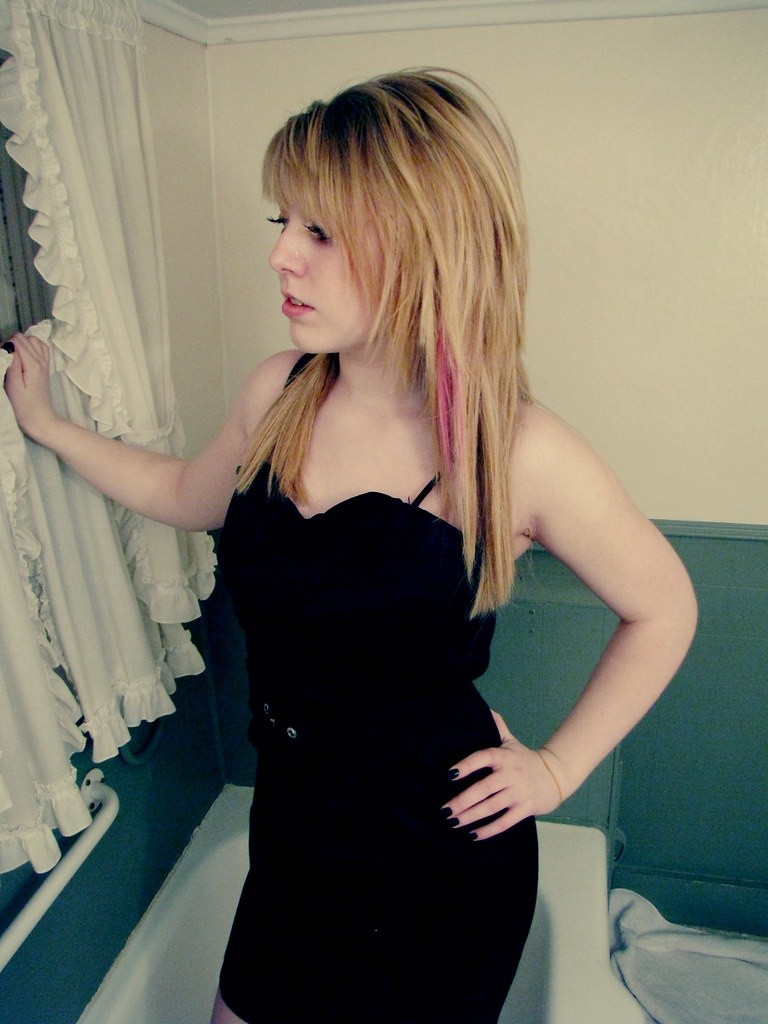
[3,68,700,1024]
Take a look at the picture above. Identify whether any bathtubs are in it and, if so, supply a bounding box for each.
[72,780,613,1024]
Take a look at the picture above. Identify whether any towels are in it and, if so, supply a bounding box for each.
[606,886,767,1023]
